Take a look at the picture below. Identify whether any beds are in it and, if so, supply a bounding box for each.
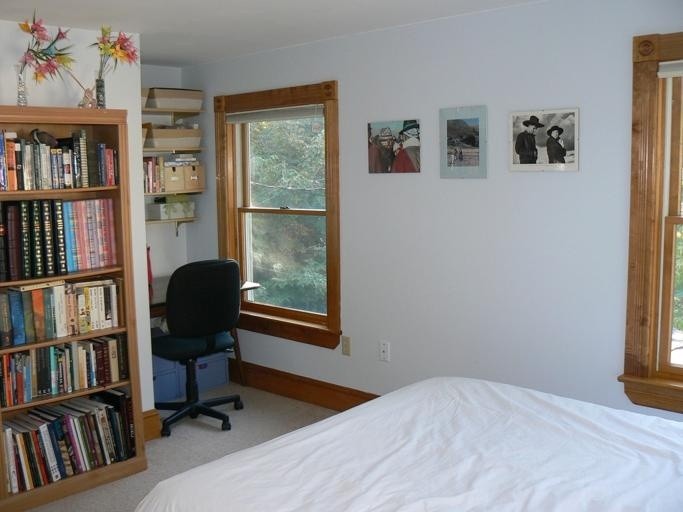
[132,371,679,511]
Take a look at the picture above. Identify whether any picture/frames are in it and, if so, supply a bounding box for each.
[509,107,579,173]
[436,106,488,181]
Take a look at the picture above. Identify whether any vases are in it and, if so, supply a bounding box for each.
[15,60,27,106]
[95,80,105,109]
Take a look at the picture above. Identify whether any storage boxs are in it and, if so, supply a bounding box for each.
[140,88,149,109]
[184,161,202,191]
[151,354,230,404]
[145,127,202,148]
[146,88,204,110]
[165,162,184,192]
[141,127,148,149]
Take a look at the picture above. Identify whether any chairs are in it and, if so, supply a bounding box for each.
[147,256,248,436]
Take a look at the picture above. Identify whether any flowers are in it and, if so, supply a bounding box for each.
[85,23,139,74]
[14,11,75,83]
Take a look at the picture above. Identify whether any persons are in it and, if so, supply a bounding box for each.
[369,120,420,172]
[546,126,566,163]
[515,115,544,164]
[447,143,463,165]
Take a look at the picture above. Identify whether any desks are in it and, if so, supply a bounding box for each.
[149,268,262,387]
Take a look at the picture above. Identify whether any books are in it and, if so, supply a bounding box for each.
[145,157,165,192]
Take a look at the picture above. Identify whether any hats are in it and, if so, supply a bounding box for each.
[399,120,419,135]
[523,116,544,127]
[547,126,563,136]
[378,127,393,139]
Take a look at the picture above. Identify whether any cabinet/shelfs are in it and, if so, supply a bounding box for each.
[144,112,205,223]
[0,103,148,512]
